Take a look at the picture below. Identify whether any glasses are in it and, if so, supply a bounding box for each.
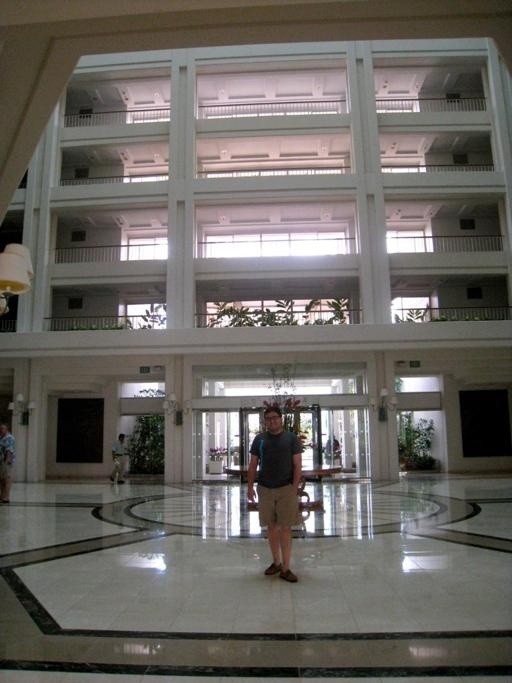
[264,415,282,421]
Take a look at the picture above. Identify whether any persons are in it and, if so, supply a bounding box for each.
[109,433,129,483]
[245,407,305,583]
[0,424,15,504]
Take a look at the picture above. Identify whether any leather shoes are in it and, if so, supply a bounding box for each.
[278,568,297,583]
[264,562,282,575]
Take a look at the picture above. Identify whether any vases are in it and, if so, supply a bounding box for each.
[208,457,226,474]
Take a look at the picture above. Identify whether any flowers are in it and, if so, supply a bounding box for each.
[205,447,227,460]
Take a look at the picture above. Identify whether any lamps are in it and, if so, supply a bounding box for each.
[367,389,396,422]
[161,392,193,427]
[0,241,38,426]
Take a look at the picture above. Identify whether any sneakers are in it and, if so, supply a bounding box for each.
[110,476,125,484]
